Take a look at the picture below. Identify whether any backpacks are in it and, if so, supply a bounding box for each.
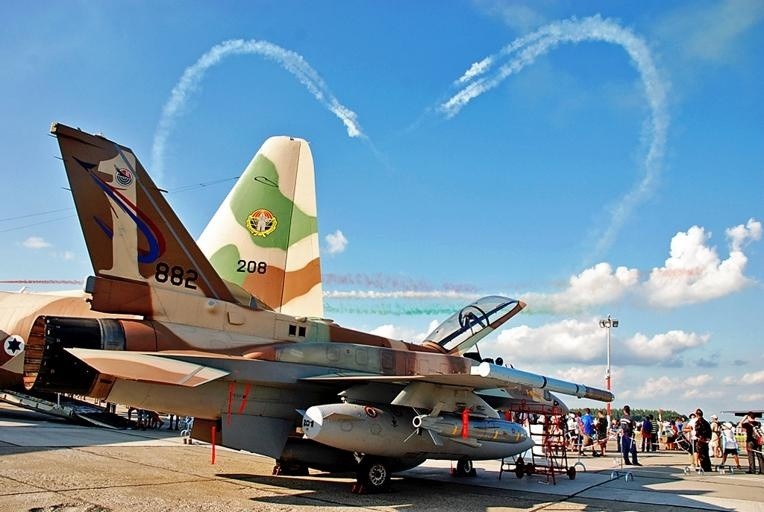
[749,422,764,446]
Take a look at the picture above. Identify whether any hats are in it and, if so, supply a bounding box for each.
[711,415,718,419]
[723,423,732,428]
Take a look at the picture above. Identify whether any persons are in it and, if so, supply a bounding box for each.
[153,414,166,431]
[497,405,764,477]
[126,406,140,421]
[135,408,148,431]
[166,414,181,433]
[148,411,155,428]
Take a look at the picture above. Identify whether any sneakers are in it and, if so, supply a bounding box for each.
[696,468,712,472]
[719,465,741,469]
[580,451,606,456]
[625,462,642,466]
[746,471,762,474]
